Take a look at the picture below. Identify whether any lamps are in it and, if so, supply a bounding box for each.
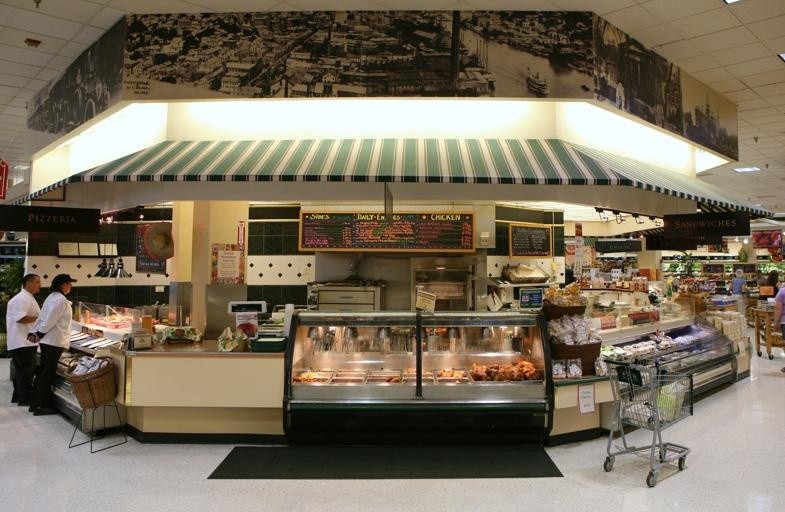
[595,207,661,227]
[98,214,115,224]
[94,224,108,277]
[102,224,116,278]
[138,206,145,220]
[110,224,131,278]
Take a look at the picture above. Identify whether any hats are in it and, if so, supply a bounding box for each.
[51,274,77,288]
[735,269,743,278]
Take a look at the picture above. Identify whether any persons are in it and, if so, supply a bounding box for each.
[773,286,785,372]
[750,270,766,284]
[6,274,41,406]
[26,274,78,415]
[758,270,778,300]
[731,269,746,294]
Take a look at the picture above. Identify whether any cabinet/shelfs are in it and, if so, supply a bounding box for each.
[10,302,126,438]
[661,259,758,299]
[282,310,554,447]
[597,300,737,431]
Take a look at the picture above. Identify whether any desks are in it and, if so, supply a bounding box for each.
[753,309,775,359]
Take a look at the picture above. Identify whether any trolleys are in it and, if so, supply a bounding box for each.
[604,359,693,487]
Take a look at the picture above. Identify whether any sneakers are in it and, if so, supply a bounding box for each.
[11,396,59,415]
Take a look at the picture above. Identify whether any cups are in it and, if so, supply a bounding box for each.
[511,300,520,312]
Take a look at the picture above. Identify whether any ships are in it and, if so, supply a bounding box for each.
[527,72,550,96]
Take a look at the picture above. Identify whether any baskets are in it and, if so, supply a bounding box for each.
[550,335,602,375]
[543,298,586,320]
[66,356,115,408]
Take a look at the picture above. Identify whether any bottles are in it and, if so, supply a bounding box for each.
[668,287,672,297]
[653,293,658,306]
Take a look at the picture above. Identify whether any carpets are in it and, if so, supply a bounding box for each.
[206,446,565,479]
